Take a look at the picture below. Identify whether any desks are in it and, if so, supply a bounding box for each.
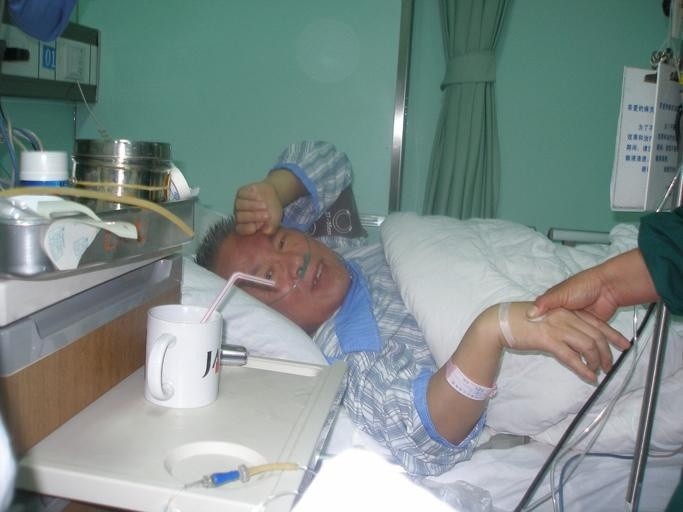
[14,355,348,512]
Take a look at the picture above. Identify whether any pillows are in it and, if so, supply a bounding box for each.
[183,255,328,377]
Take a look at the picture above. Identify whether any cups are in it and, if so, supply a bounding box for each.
[144,304,223,410]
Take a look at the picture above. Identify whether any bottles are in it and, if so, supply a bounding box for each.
[19,150,68,202]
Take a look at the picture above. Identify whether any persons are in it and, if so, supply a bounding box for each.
[191,137,638,483]
[525,194,683,326]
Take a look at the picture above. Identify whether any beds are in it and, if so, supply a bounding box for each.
[317,405,682,512]
